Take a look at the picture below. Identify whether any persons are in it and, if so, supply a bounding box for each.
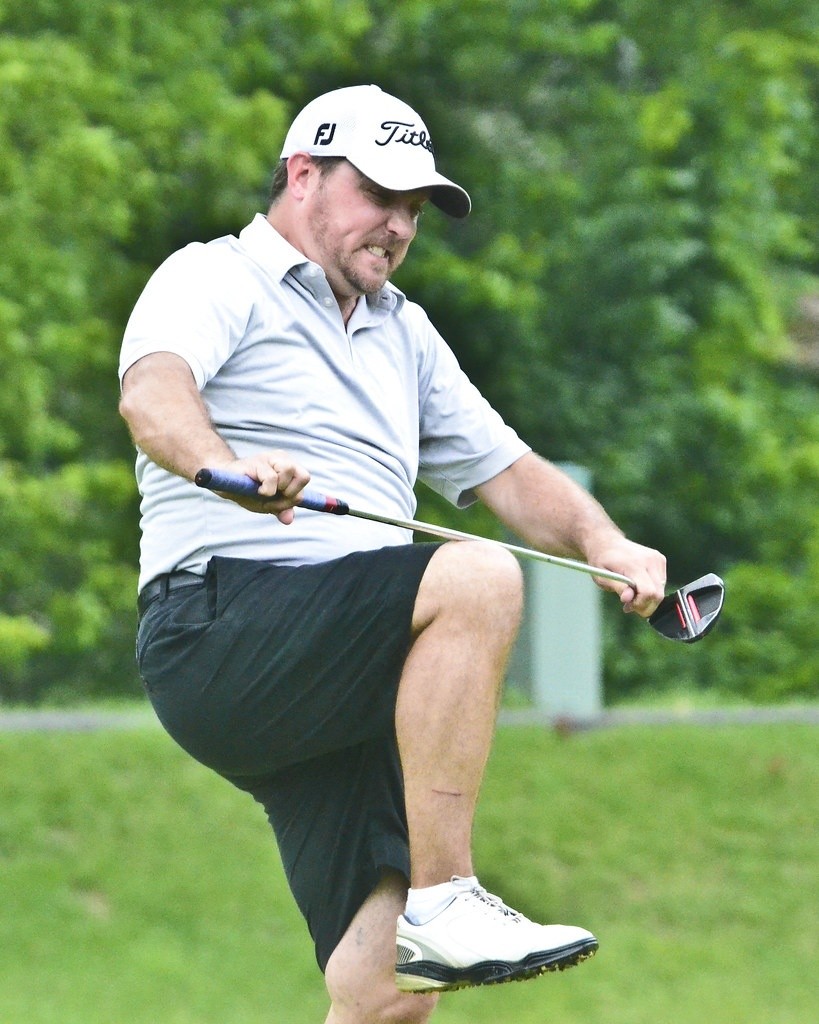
[118,83,668,1023]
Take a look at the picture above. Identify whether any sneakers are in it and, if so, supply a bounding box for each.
[394,875,600,993]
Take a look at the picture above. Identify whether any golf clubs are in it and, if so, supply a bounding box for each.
[195,467,726,643]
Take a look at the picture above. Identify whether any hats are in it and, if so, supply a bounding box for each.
[279,84,470,219]
